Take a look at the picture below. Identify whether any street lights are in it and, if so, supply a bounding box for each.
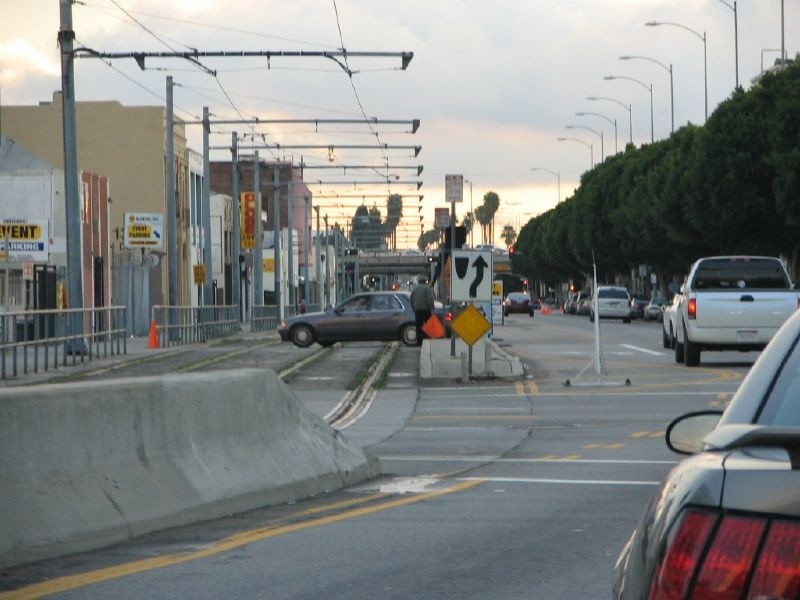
[565,123,605,164]
[643,20,708,123]
[576,111,618,152]
[530,166,561,204]
[604,74,655,144]
[618,54,675,133]
[588,95,633,145]
[558,135,595,170]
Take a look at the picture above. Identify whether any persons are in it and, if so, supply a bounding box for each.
[410,274,435,345]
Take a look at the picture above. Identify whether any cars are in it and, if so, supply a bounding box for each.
[563,283,669,323]
[660,254,800,367]
[501,292,536,319]
[609,305,800,600]
[275,290,454,347]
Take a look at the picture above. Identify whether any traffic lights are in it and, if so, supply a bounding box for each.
[508,247,516,258]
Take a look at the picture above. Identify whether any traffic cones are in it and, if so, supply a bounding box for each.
[145,321,159,350]
